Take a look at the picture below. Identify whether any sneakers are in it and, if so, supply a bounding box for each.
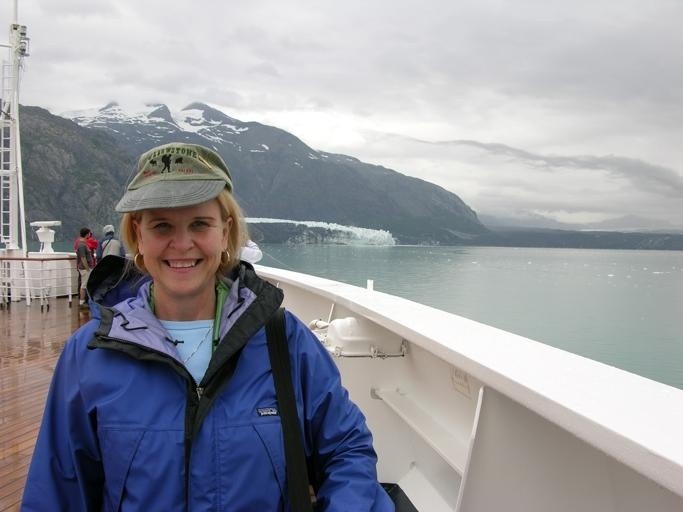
[79,302,90,309]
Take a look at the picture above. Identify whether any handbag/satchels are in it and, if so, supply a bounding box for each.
[283,482,419,512]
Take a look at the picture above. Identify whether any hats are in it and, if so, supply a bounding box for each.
[103,225,115,234]
[115,142,234,213]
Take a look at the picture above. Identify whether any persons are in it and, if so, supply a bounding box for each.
[20,142,395,512]
[74,225,125,309]
